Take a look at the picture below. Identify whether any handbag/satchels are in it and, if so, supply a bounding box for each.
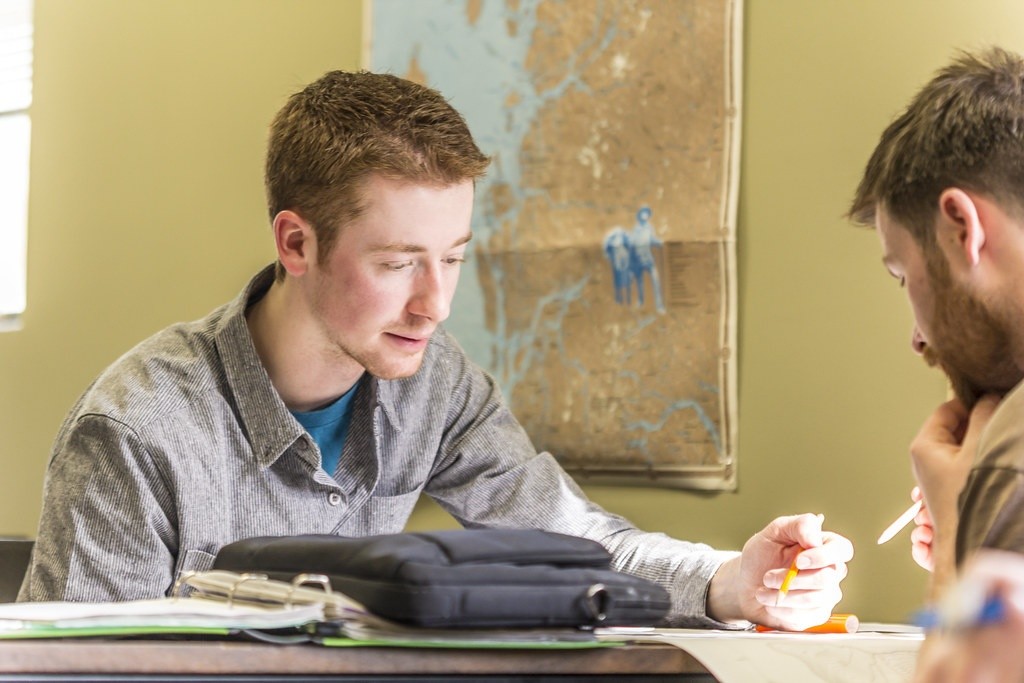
[209,527,672,626]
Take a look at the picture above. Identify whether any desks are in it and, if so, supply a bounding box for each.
[0,626,924,683]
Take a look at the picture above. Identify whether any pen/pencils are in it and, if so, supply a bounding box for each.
[910,596,1002,627]
[877,497,926,546]
[775,513,826,606]
[746,613,859,634]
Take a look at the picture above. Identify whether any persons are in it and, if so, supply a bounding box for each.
[842,44,1024,626]
[11,68,855,633]
